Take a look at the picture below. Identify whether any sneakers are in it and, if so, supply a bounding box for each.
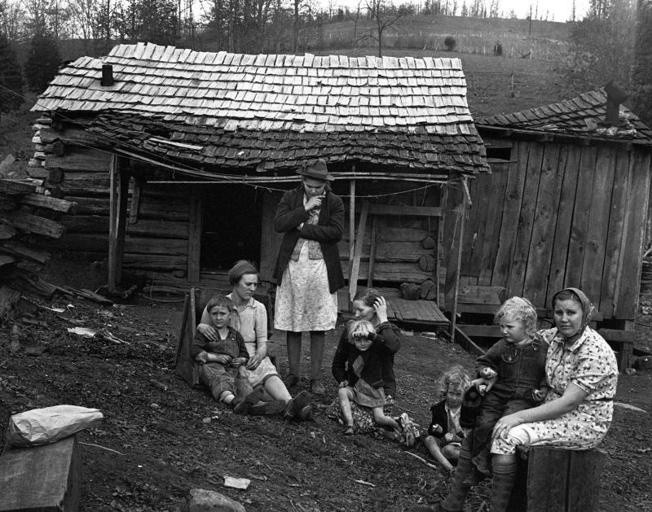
[403,503,449,512]
[234,374,326,421]
[463,455,490,486]
[345,425,357,434]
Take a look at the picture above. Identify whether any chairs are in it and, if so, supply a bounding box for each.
[174,288,282,390]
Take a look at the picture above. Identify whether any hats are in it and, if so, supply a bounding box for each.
[296,159,335,181]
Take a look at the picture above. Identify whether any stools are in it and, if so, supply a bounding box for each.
[516,446,608,512]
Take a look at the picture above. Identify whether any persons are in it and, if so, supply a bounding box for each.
[325,288,421,447]
[195,259,314,421]
[462,296,549,487]
[423,365,472,473]
[440,286,619,512]
[272,158,346,395]
[189,294,286,416]
[338,319,403,436]
